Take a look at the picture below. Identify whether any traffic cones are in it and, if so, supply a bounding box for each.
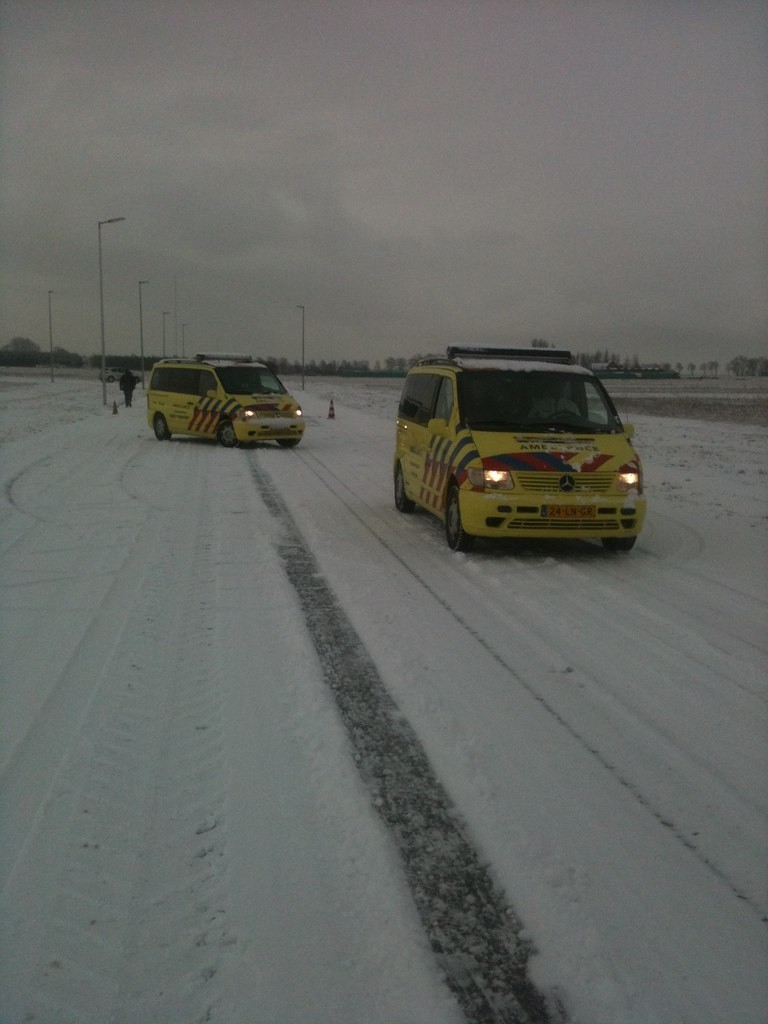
[113,400,118,415]
[328,399,336,420]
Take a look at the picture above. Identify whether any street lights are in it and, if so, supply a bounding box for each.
[181,322,189,358]
[163,311,172,360]
[47,288,58,384]
[136,280,149,391]
[95,214,126,405]
[296,304,306,391]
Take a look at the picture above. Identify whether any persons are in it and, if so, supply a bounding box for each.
[528,381,582,419]
[120,369,136,407]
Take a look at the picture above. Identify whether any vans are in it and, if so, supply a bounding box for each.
[392,346,648,552]
[145,352,306,447]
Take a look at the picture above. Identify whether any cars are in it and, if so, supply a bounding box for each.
[99,366,141,385]
